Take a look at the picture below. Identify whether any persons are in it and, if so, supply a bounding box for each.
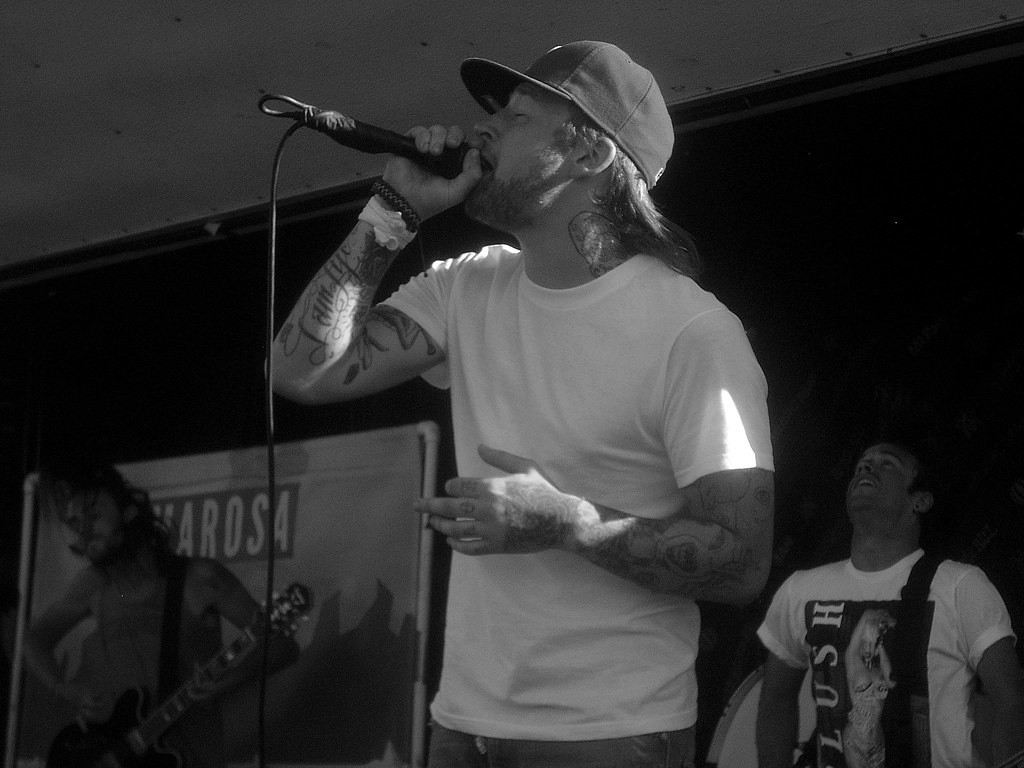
[22,463,298,768]
[757,436,1024,768]
[262,42,778,768]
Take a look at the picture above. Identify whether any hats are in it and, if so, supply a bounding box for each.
[459,41,675,193]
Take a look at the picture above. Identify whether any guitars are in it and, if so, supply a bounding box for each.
[46,582,315,768]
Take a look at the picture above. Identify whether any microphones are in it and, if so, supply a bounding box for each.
[300,106,473,179]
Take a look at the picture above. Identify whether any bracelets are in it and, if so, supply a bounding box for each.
[358,179,421,252]
